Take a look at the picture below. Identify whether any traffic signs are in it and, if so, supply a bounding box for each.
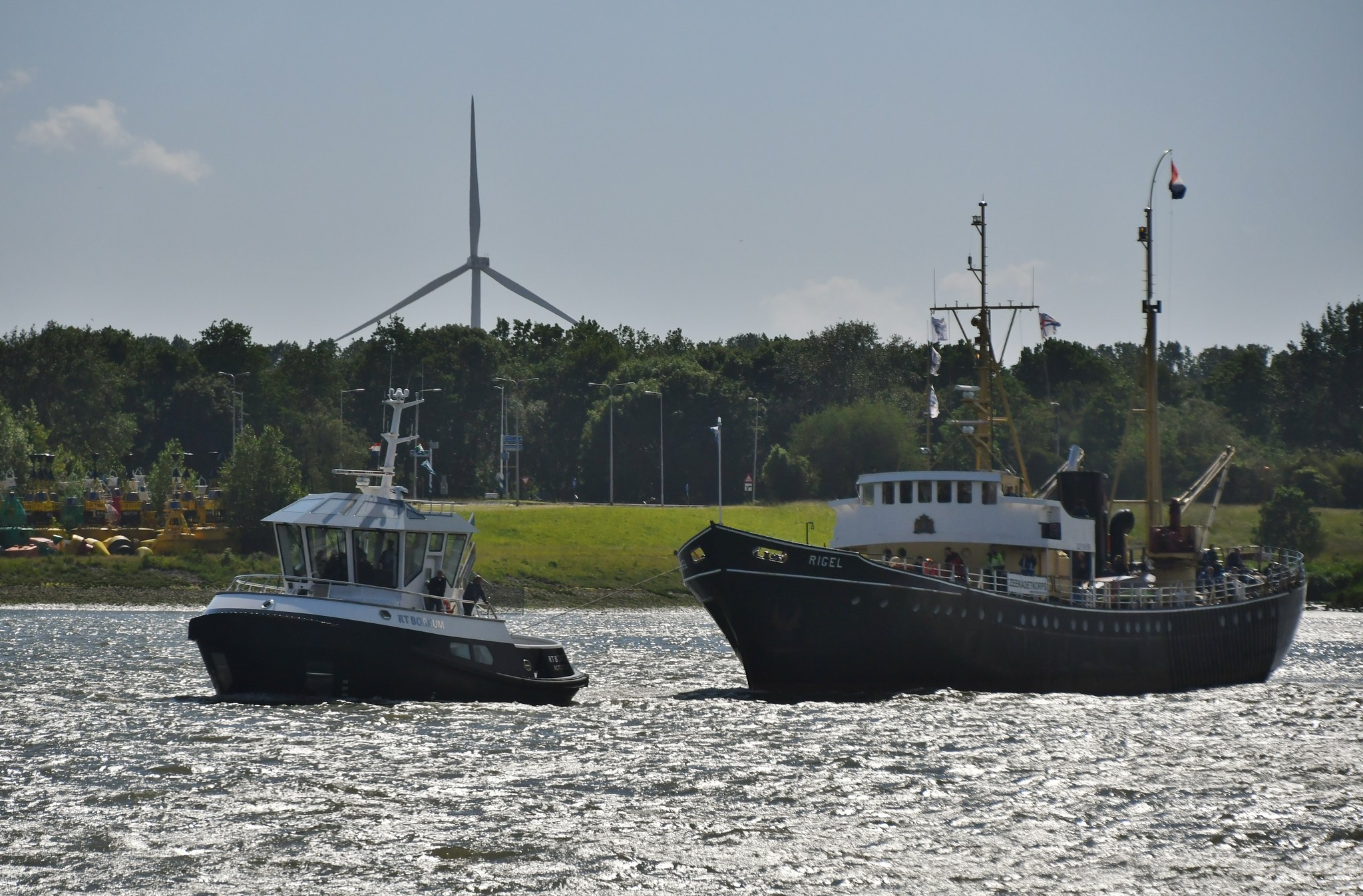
[502,444,523,451]
[503,435,523,443]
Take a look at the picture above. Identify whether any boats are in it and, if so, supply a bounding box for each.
[671,146,1306,704]
[186,387,594,707]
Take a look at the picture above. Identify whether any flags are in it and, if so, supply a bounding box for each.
[1168,161,1187,199]
[931,316,948,344]
[929,384,940,418]
[1039,313,1061,339]
[930,347,942,377]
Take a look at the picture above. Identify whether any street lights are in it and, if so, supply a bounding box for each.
[218,370,251,457]
[645,391,665,507]
[492,377,540,508]
[1050,402,1061,469]
[588,381,637,505]
[339,388,364,479]
[413,388,441,499]
[748,397,759,506]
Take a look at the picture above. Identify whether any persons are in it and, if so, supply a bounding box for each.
[1073,550,1127,605]
[1019,547,1038,578]
[316,549,348,586]
[428,569,446,612]
[1196,543,1295,603]
[358,552,374,585]
[943,546,967,585]
[884,547,907,566]
[985,544,1007,594]
[913,556,923,573]
[380,539,394,588]
[1002,485,1019,497]
[462,575,489,616]
